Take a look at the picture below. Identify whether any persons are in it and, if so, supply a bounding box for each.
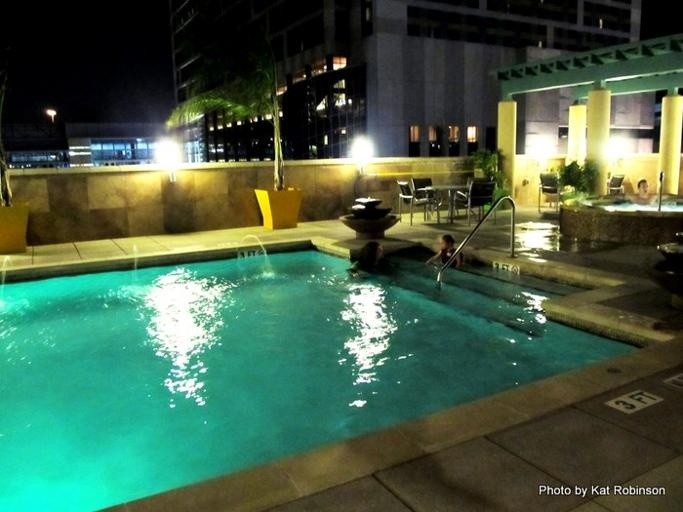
[345,241,384,280]
[598,180,682,207]
[424,234,465,270]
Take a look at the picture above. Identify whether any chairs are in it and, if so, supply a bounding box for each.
[607,175,625,195]
[397,176,497,228]
[538,174,565,214]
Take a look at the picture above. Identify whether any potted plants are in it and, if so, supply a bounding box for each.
[167,58,307,231]
[0,70,32,255]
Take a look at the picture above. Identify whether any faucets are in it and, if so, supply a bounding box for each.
[657,171,664,211]
